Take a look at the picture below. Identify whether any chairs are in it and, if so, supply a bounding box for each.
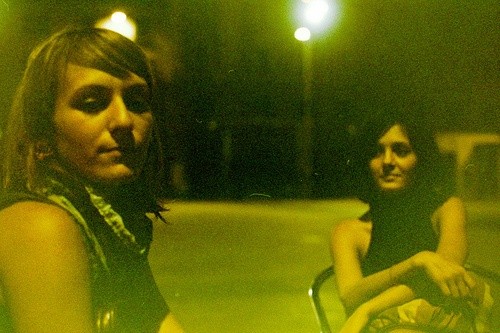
[308,259,499,333]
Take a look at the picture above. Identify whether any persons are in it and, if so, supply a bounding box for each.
[0,25,172,332]
[327,100,495,333]
